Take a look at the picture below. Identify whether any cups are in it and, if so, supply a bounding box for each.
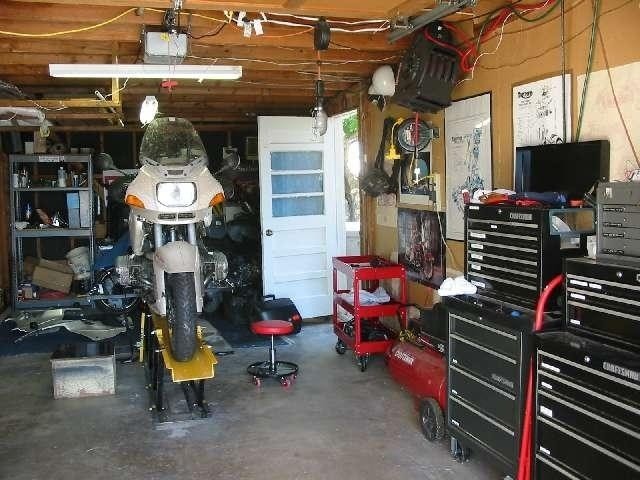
[25,142,35,156]
[33,131,47,154]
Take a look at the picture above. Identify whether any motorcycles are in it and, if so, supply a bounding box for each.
[92,117,240,362]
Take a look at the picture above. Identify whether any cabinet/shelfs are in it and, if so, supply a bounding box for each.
[6,151,96,313]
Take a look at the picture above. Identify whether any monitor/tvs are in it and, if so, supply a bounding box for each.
[515,139,610,206]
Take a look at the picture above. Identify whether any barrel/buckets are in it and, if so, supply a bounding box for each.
[67,247,91,280]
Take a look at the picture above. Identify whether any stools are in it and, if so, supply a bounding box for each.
[247,319,299,387]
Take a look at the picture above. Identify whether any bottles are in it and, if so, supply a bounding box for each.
[58,167,66,189]
